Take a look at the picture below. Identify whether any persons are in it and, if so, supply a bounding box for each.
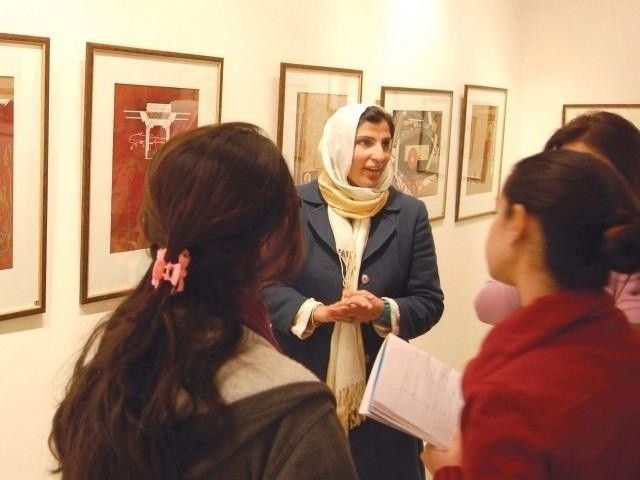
[254,103,445,480]
[46,122,364,480]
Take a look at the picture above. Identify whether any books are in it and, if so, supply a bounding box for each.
[358,332,465,450]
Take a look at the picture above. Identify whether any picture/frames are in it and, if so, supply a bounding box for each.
[276,61,508,224]
[1,32,51,321]
[78,41,222,304]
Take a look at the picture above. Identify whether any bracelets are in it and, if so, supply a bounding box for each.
[311,304,323,328]
